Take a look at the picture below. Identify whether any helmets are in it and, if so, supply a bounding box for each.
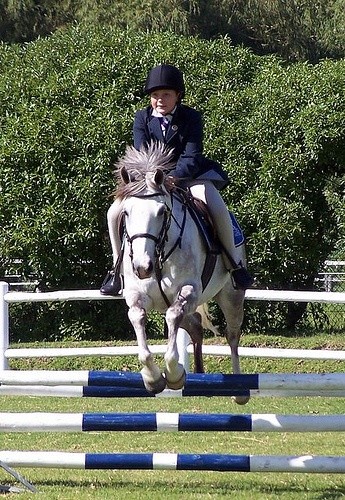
[144,64,185,97]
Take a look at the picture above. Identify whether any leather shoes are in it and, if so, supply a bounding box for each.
[100,275,121,295]
[231,266,253,288]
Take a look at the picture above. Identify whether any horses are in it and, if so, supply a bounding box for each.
[103,136,253,406]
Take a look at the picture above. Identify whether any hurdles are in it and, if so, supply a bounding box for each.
[0,279,345,489]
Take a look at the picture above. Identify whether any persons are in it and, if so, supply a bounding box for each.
[100,64,255,296]
